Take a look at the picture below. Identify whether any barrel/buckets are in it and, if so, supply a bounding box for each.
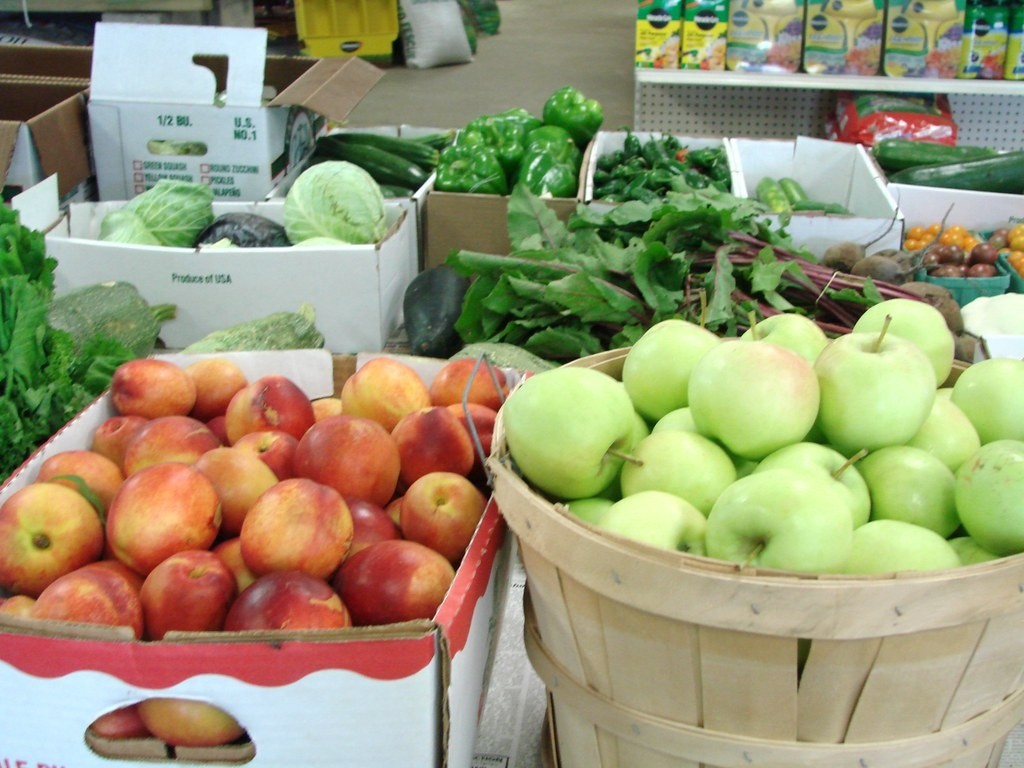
[458,345,1024,768]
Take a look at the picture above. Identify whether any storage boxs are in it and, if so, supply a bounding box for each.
[0,0,1024,768]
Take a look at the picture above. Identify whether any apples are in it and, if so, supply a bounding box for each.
[503,298,1023,575]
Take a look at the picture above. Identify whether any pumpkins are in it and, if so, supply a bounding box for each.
[47,279,325,359]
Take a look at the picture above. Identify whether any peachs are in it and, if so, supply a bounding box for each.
[0,356,506,748]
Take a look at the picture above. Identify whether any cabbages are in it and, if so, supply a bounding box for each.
[96,161,386,247]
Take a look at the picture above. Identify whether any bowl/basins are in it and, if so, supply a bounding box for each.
[914,228,1009,311]
[980,229,1023,294]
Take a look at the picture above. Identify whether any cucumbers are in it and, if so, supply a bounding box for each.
[756,138,1024,214]
[304,128,457,198]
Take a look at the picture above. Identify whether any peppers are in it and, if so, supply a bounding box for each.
[437,86,732,203]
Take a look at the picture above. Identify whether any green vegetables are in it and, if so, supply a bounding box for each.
[443,178,930,365]
[0,194,136,484]
